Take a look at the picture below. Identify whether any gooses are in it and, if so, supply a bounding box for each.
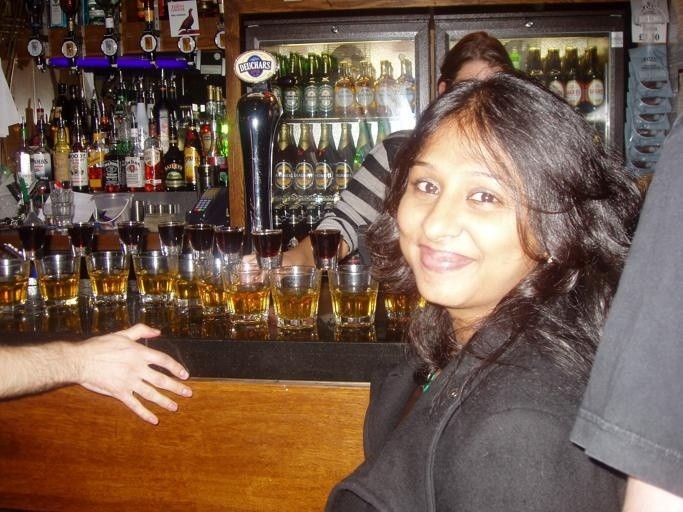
[178,8,194,32]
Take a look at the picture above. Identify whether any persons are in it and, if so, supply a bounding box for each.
[570,113,683,512]
[238,31,514,284]
[324,72,645,512]
[0,323,192,427]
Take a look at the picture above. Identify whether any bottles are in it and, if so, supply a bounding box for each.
[8,68,227,224]
[494,46,607,117]
[256,51,417,250]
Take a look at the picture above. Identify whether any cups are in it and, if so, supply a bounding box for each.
[1,255,31,314]
[221,262,271,327]
[327,263,380,333]
[117,223,147,277]
[155,257,192,315]
[310,228,341,275]
[267,265,321,337]
[216,227,244,263]
[133,249,166,305]
[187,226,216,261]
[68,224,97,272]
[384,275,425,322]
[157,223,185,256]
[253,229,285,269]
[18,225,49,262]
[34,254,81,307]
[193,258,230,318]
[85,250,130,307]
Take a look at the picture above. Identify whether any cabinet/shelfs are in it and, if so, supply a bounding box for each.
[433,14,626,173]
[242,14,435,242]
